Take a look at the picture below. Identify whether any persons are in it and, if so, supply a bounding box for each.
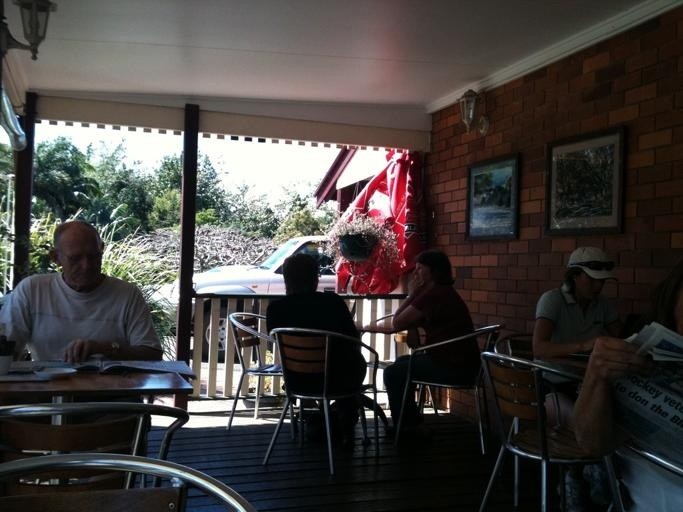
[265,253,367,451]
[530,245,620,511]
[362,247,482,439]
[561,258,683,511]
[1,221,165,364]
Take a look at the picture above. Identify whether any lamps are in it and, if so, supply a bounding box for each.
[457,83,491,139]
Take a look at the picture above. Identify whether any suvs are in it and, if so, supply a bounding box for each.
[186,235,336,362]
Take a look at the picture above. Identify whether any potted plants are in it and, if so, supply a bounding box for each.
[316,209,402,294]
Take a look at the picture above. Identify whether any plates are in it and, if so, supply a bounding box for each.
[5,366,43,373]
[32,368,76,379]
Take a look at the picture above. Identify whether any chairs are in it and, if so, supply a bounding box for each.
[225,309,305,444]
[0,399,190,488]
[0,447,258,511]
[387,324,502,458]
[478,329,683,512]
[360,313,399,369]
[262,328,380,474]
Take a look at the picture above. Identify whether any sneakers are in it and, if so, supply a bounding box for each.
[384,412,425,441]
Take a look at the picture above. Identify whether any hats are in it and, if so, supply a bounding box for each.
[566,245,620,283]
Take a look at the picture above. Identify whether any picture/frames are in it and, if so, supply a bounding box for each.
[463,153,523,243]
[543,122,626,237]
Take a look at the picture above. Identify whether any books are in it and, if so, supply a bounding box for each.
[7,359,197,380]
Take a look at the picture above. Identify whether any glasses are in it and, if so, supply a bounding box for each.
[568,260,616,272]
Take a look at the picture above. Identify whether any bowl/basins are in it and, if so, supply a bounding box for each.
[0,355,13,375]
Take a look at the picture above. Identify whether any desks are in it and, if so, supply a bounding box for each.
[0,360,198,483]
[292,324,401,448]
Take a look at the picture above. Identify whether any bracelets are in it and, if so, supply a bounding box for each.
[111,339,134,359]
[405,293,413,300]
